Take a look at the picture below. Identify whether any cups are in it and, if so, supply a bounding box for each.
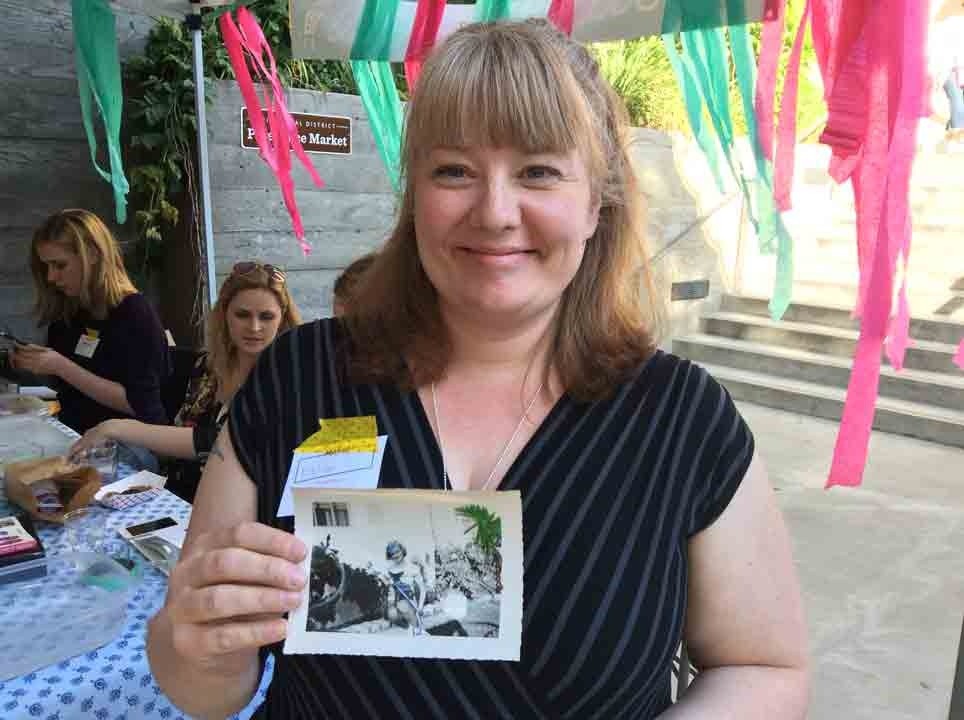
[88,440,118,486]
[63,506,107,555]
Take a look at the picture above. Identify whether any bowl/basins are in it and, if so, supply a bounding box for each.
[0,551,140,681]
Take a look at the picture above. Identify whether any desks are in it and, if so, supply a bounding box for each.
[0,379,275,720]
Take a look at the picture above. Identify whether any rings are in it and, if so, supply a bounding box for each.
[84,433,90,440]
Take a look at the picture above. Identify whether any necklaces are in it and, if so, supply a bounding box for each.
[432,368,551,491]
[215,381,241,404]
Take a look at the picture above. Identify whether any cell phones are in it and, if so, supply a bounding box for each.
[0,331,30,345]
[116,515,180,541]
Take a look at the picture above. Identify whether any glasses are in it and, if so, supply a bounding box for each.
[236,262,285,282]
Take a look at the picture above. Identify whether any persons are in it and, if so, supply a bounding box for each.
[147,18,811,720]
[9,209,173,475]
[332,254,379,316]
[383,541,425,627]
[66,264,303,505]
